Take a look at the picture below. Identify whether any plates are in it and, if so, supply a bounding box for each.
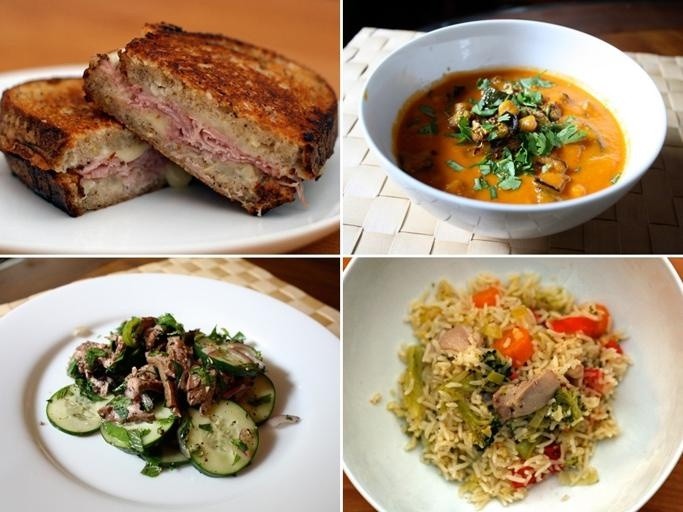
[0,46,340,254]
[1,274,342,512]
[341,259,683,512]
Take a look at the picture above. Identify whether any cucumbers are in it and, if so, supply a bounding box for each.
[46,335,276,478]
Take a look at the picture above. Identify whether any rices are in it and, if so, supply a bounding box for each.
[369,270,636,510]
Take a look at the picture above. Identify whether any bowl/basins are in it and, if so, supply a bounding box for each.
[365,18,666,241]
